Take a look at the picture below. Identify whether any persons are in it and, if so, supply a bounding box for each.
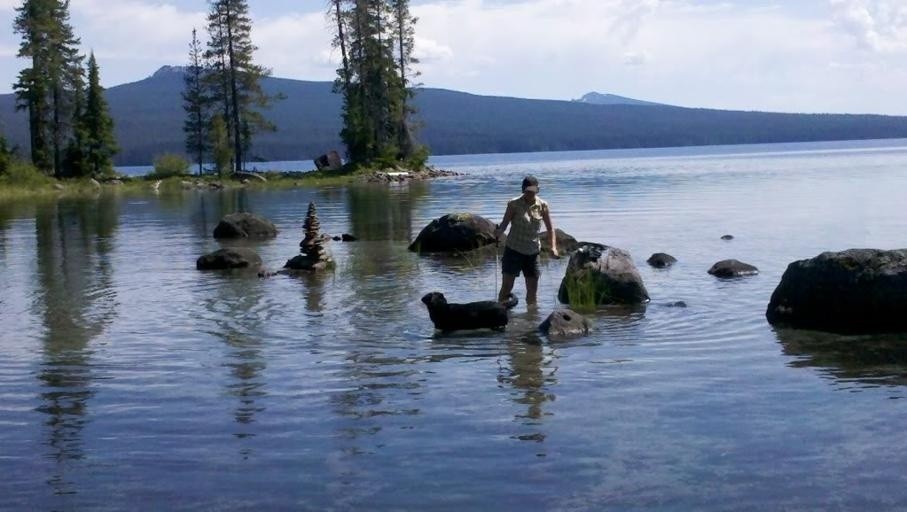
[494,177,559,304]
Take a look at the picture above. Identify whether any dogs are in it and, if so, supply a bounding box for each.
[422,290,521,336]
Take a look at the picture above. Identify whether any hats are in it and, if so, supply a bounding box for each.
[522,176,539,193]
